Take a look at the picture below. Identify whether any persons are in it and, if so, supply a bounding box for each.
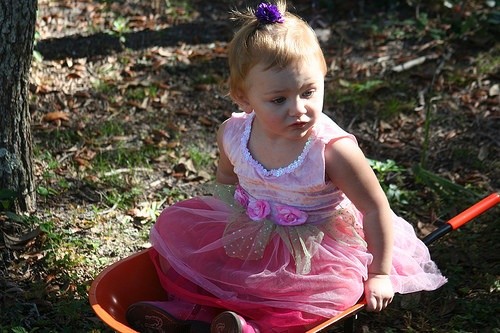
[125,0,450,333]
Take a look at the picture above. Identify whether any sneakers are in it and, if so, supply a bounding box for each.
[125,296,215,329]
[214,308,258,333]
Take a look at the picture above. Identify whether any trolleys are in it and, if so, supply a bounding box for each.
[82,191,500,333]
